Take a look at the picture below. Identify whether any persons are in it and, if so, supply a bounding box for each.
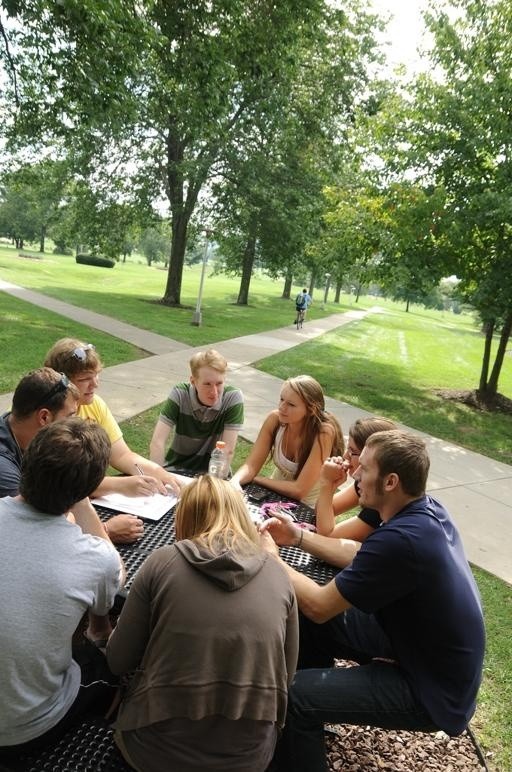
[0,415,122,761]
[45,338,183,498]
[231,375,345,509]
[315,417,398,542]
[294,289,312,324]
[259,428,487,772]
[105,476,300,772]
[0,366,145,654]
[150,348,243,474]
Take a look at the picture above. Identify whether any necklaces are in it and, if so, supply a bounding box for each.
[285,425,289,457]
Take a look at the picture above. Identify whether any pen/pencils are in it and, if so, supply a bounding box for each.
[134,464,144,476]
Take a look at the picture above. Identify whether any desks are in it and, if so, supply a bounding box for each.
[85,470,343,625]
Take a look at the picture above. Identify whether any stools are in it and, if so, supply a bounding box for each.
[28,712,138,771]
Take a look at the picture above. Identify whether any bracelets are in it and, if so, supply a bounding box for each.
[297,529,304,548]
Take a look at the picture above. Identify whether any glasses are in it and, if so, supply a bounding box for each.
[70,344,96,361]
[42,372,68,401]
[348,448,360,456]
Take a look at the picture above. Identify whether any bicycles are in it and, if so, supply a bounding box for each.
[297,307,305,330]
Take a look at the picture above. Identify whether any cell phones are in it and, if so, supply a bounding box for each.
[248,491,269,502]
